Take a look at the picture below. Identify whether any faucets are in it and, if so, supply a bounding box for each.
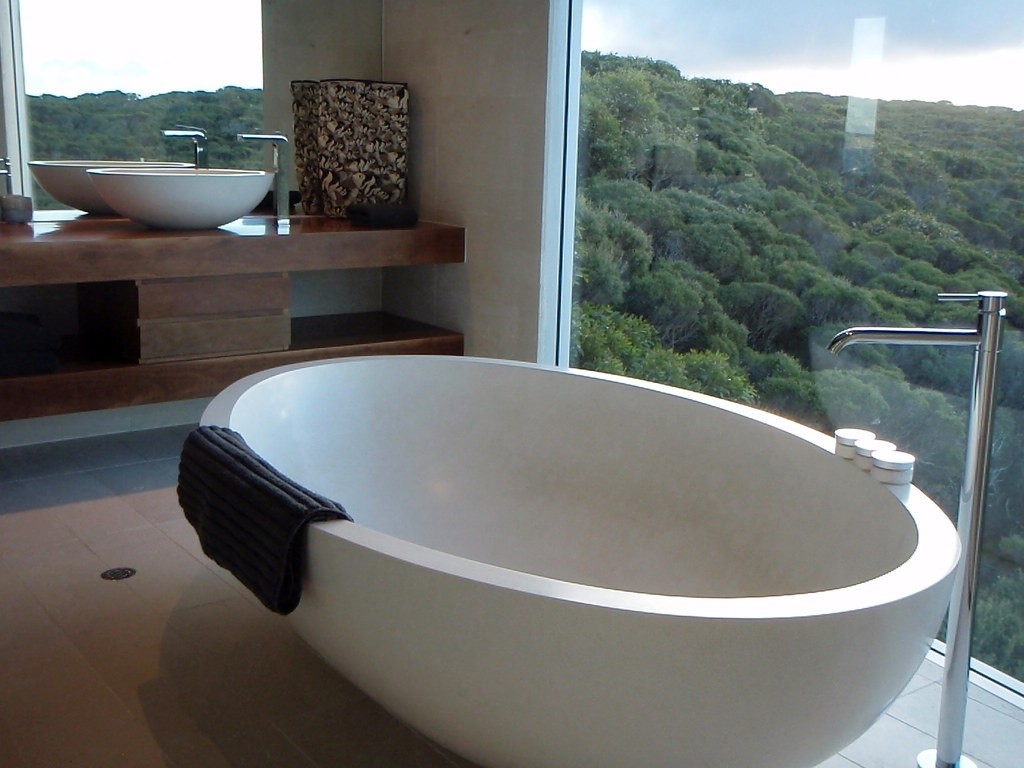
[159,126,208,167]
[823,328,978,358]
[236,128,290,217]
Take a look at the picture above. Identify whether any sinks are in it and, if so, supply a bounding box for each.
[85,168,275,230]
[27,161,197,214]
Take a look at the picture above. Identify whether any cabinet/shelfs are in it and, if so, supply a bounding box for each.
[0,215,465,422]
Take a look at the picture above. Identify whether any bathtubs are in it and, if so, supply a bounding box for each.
[198,354,964,768]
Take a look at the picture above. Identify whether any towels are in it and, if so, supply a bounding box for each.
[345,204,418,228]
[177,425,356,616]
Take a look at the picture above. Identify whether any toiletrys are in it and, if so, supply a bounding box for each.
[835,428,916,485]
